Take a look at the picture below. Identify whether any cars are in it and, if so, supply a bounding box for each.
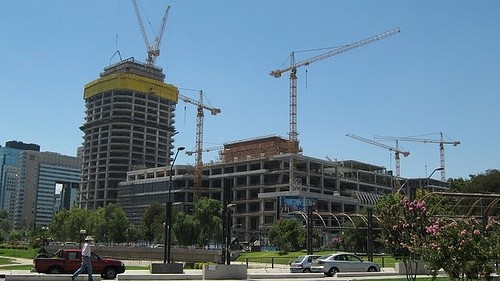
[310,253,380,277]
[148,244,172,249]
[290,255,322,273]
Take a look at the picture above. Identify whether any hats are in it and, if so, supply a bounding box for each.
[85,236,94,240]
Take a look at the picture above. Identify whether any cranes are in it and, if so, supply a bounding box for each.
[132,0,171,67]
[150,83,224,207]
[269,27,402,156]
[374,131,461,187]
[346,131,411,193]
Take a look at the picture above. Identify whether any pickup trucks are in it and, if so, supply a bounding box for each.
[34,249,125,279]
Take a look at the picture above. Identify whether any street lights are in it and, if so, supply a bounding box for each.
[76,229,87,250]
[422,168,444,197]
[163,146,186,262]
[41,226,49,254]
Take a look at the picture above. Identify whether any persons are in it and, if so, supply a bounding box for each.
[71,236,94,281]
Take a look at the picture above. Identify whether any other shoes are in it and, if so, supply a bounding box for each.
[71,276,74,281]
